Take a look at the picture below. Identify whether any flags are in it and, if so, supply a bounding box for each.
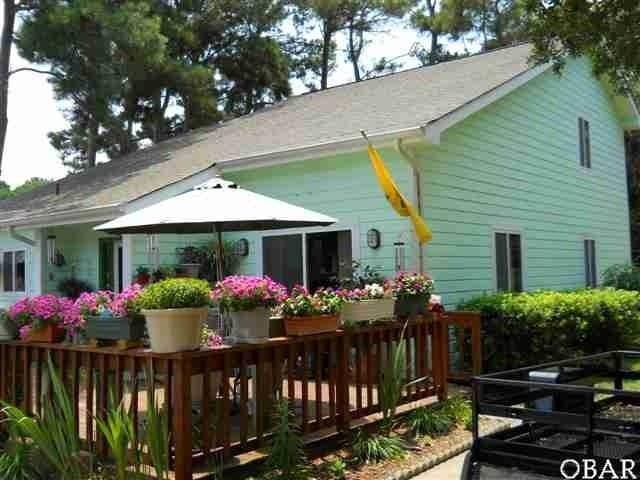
[359,132,437,245]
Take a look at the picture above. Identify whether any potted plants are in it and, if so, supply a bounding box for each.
[135,246,204,282]
[134,278,210,354]
[0,307,17,342]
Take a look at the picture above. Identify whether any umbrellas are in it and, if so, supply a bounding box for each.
[91,173,338,336]
